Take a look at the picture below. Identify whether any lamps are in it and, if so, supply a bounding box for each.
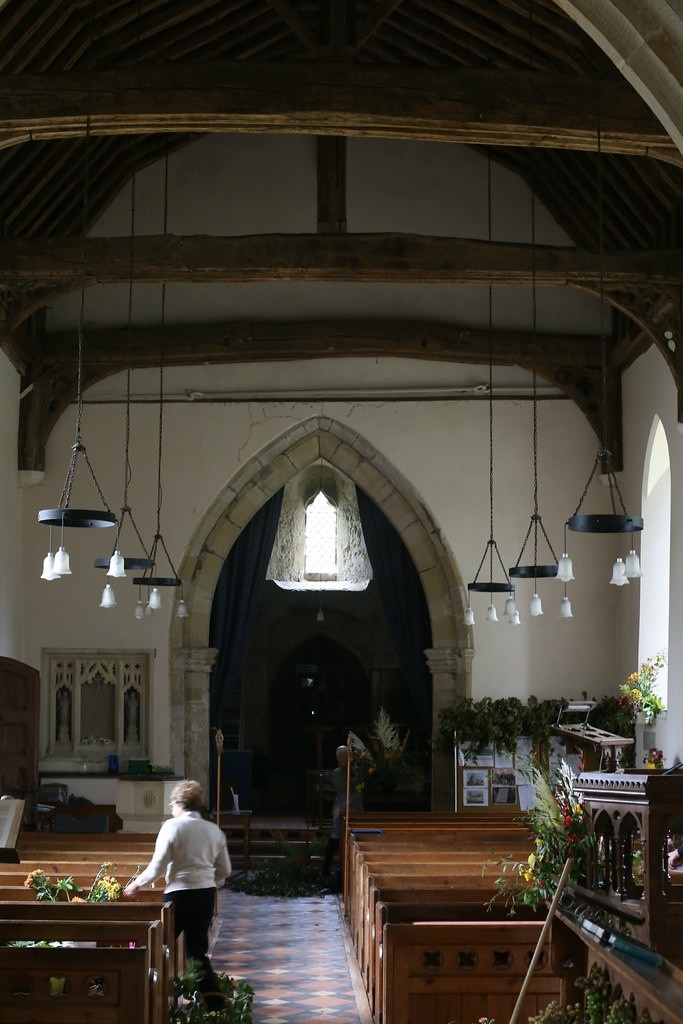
[459,53,651,634]
[33,34,195,625]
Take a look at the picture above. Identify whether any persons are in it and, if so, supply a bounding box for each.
[124,778,232,983]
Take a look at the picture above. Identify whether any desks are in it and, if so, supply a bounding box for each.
[216,806,254,881]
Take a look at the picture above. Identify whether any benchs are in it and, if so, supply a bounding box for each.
[0,826,188,1024]
[341,804,565,1024]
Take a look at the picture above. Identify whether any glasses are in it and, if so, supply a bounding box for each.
[167,800,183,810]
